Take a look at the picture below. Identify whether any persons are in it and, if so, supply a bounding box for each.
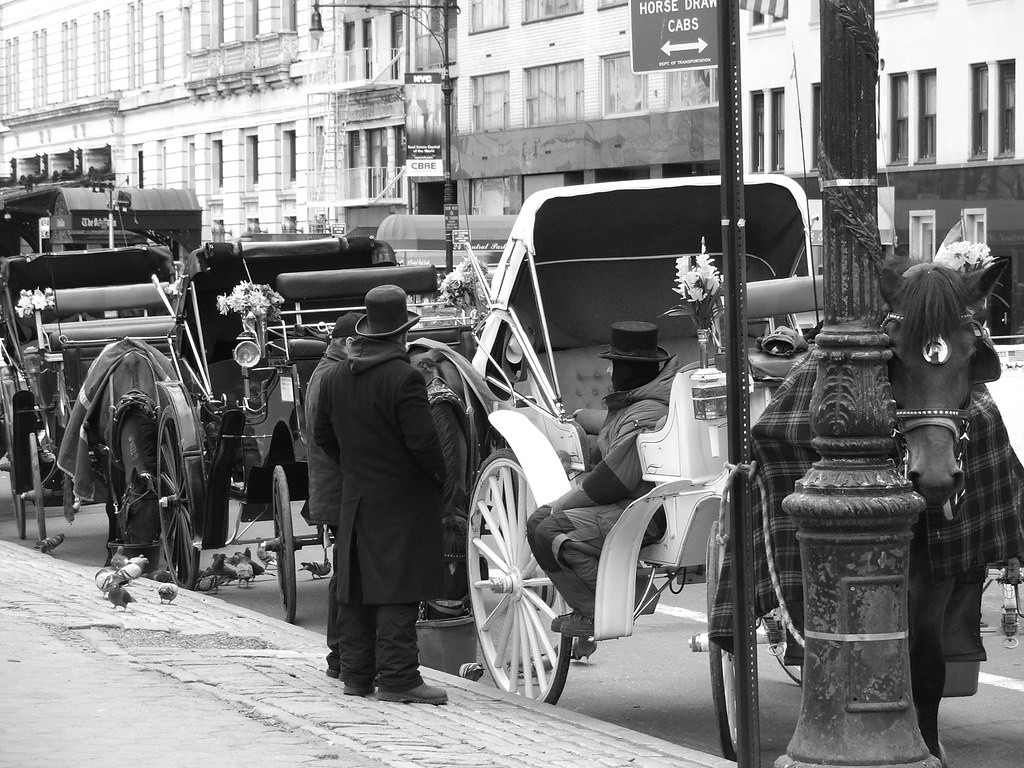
[314,284,449,703]
[305,313,381,684]
[527,321,681,635]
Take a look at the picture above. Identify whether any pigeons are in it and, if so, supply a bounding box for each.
[157,583,178,605]
[298,561,332,580]
[33,533,66,555]
[458,662,486,683]
[150,569,173,583]
[257,538,277,570]
[104,583,138,612]
[195,548,266,596]
[505,634,597,679]
[110,546,149,587]
[95,569,129,598]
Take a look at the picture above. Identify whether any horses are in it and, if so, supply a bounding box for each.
[405,348,473,620]
[90,349,161,571]
[881,259,1011,768]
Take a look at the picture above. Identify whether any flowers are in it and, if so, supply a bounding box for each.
[217,281,285,323]
[165,280,182,297]
[657,236,726,329]
[14,286,56,319]
[933,240,997,273]
[438,255,489,309]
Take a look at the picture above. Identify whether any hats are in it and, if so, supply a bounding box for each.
[354,285,422,338]
[596,321,671,361]
[332,312,365,339]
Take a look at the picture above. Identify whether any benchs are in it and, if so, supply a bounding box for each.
[39,281,177,362]
[538,331,763,471]
[14,338,50,361]
[266,265,474,362]
[747,274,823,392]
[194,359,272,447]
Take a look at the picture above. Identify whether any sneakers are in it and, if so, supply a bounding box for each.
[345,680,376,696]
[378,676,447,704]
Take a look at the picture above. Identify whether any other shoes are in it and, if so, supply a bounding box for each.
[551,612,582,632]
[326,666,340,679]
[560,614,594,636]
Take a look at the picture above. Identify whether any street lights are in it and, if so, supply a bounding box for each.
[307,0,461,272]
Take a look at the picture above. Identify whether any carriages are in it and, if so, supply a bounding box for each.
[0,165,478,621]
[468,173,1023,761]
